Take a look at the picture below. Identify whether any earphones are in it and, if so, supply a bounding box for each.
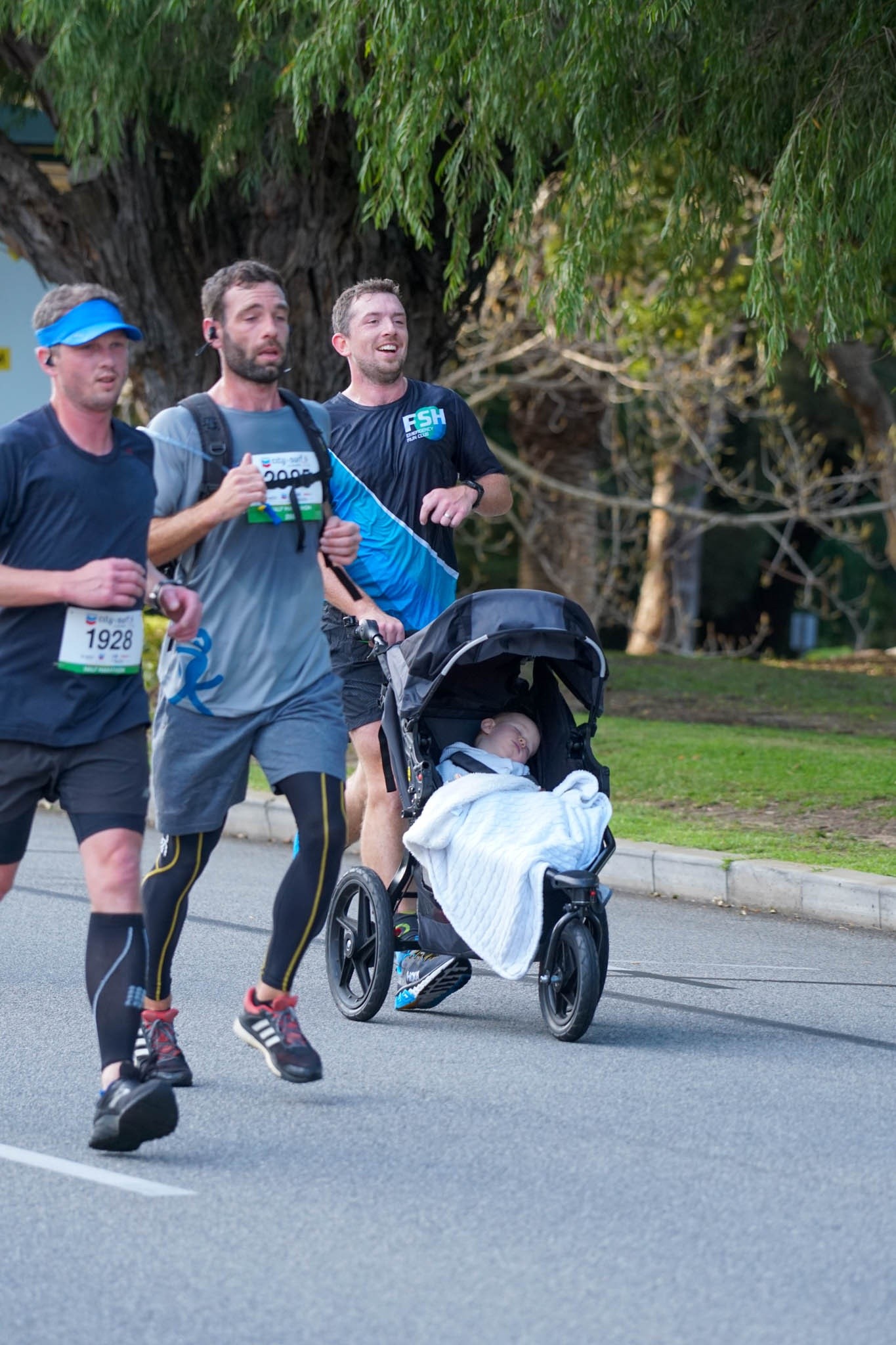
[208,327,217,339]
[44,351,54,368]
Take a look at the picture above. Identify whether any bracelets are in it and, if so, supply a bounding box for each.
[459,480,484,510]
[148,579,178,610]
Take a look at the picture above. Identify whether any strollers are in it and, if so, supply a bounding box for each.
[324,591,616,1041]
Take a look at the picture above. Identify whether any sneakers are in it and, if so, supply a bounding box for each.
[233,988,325,1083]
[88,1062,181,1154]
[132,1008,194,1086]
[392,934,473,1012]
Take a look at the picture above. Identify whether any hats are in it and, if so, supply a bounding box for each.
[35,297,144,347]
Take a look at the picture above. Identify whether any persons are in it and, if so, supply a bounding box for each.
[292,277,514,1010]
[133,259,362,1088]
[435,711,542,786]
[0,282,203,1153]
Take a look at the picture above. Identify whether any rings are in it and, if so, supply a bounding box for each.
[444,513,452,520]
